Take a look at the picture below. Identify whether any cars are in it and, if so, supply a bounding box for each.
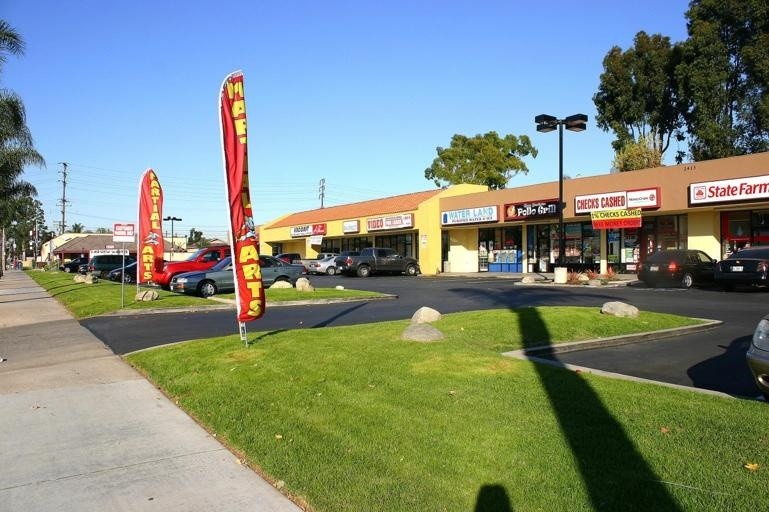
[59,245,341,297]
[638,246,768,394]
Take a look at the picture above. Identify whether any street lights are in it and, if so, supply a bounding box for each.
[163,216,182,246]
[535,113,588,283]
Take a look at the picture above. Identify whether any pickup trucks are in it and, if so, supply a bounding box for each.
[336,247,420,277]
